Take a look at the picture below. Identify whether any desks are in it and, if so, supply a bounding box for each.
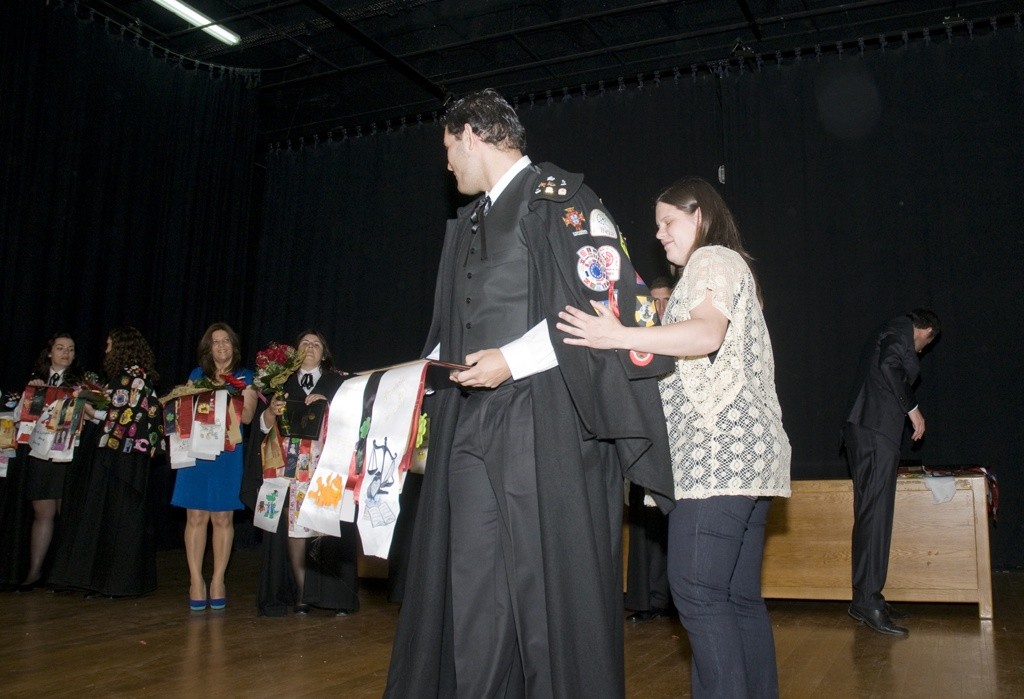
[748,470,994,618]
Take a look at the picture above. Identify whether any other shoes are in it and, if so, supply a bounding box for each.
[210,584,226,608]
[14,573,48,592]
[188,584,208,610]
[297,604,310,614]
[336,609,348,616]
[85,591,137,599]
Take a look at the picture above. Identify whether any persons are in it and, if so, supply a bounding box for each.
[625,277,675,622]
[844,309,943,637]
[557,176,792,699]
[10,324,359,618]
[383,89,673,699]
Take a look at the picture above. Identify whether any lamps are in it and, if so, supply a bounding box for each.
[154,0,241,47]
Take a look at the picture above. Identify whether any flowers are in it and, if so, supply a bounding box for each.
[259,340,307,437]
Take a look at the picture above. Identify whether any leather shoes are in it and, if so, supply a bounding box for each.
[886,602,910,617]
[627,610,657,620]
[848,603,909,635]
